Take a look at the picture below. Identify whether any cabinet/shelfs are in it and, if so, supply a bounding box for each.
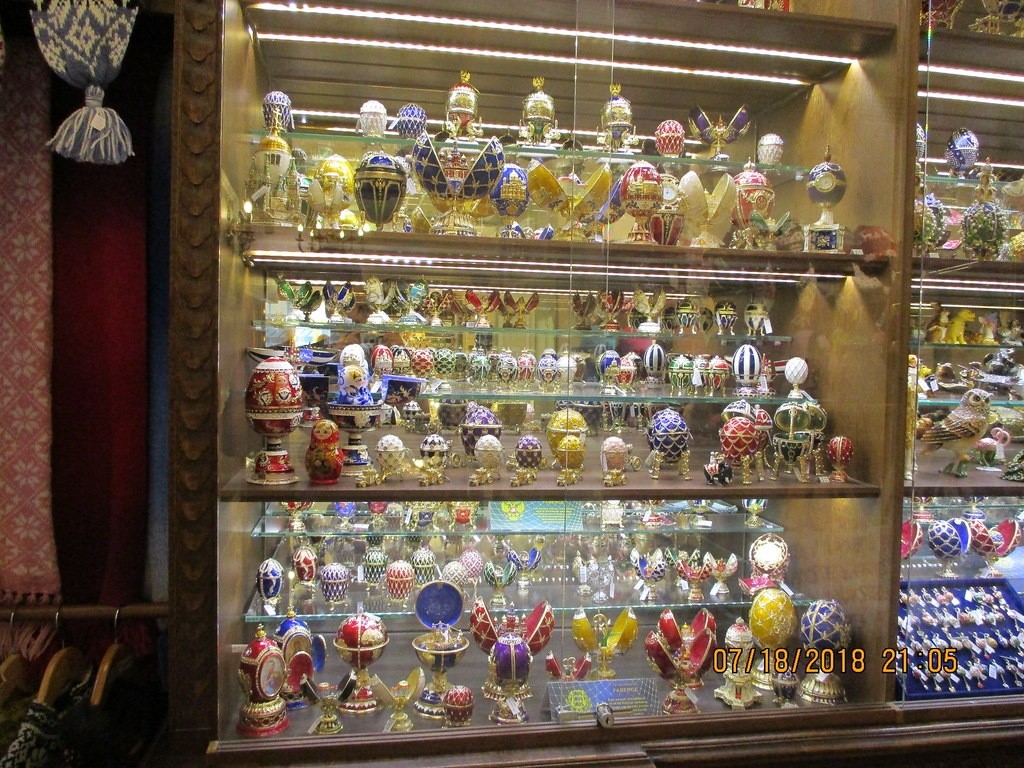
[206,1,1023,767]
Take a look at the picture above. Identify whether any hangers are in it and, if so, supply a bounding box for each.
[0,604,42,710]
[37,605,90,706]
[89,607,147,707]
